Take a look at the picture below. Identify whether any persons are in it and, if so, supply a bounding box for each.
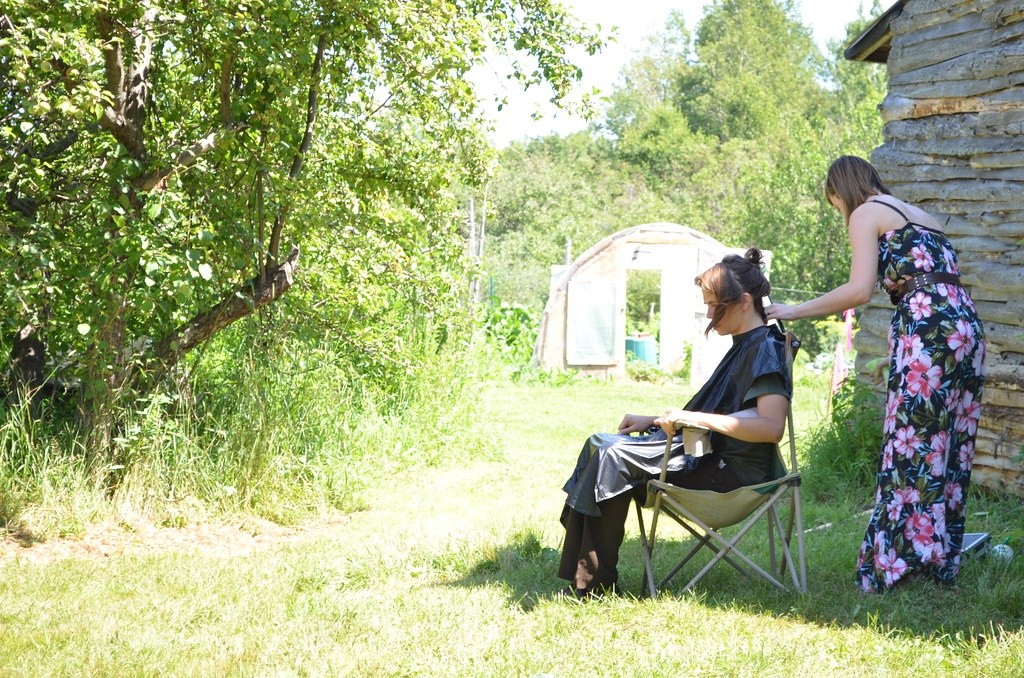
[763,156,987,598]
[556,247,801,602]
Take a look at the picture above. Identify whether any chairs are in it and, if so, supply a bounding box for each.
[624,329,808,604]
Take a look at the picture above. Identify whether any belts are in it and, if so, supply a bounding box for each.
[895,271,963,301]
